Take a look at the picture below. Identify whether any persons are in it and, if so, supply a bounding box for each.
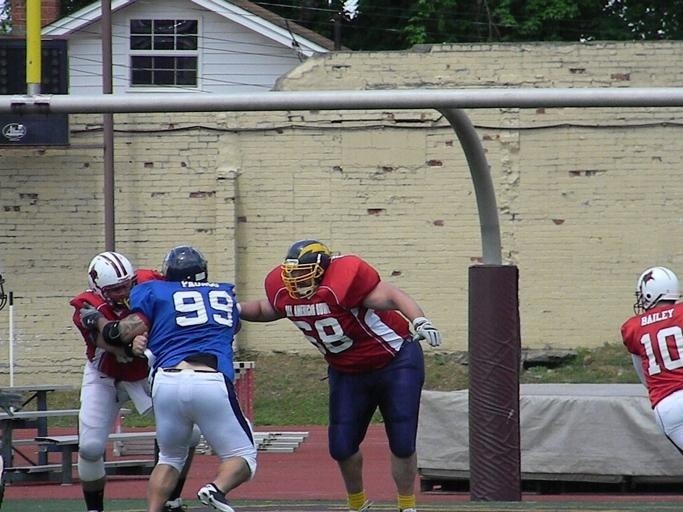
[622,265,682,458]
[125,244,257,512]
[234,240,440,511]
[68,251,202,512]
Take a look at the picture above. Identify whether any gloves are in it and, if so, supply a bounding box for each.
[77,302,102,332]
[411,315,441,348]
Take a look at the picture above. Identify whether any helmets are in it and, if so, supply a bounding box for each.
[636,266,680,310]
[86,252,137,302]
[162,246,208,283]
[285,239,331,285]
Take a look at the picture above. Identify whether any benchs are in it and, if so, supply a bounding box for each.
[0,406,131,481]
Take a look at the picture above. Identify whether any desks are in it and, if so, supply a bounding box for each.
[0,385,69,464]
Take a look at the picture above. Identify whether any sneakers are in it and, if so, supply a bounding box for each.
[196,482,234,512]
[166,498,187,512]
